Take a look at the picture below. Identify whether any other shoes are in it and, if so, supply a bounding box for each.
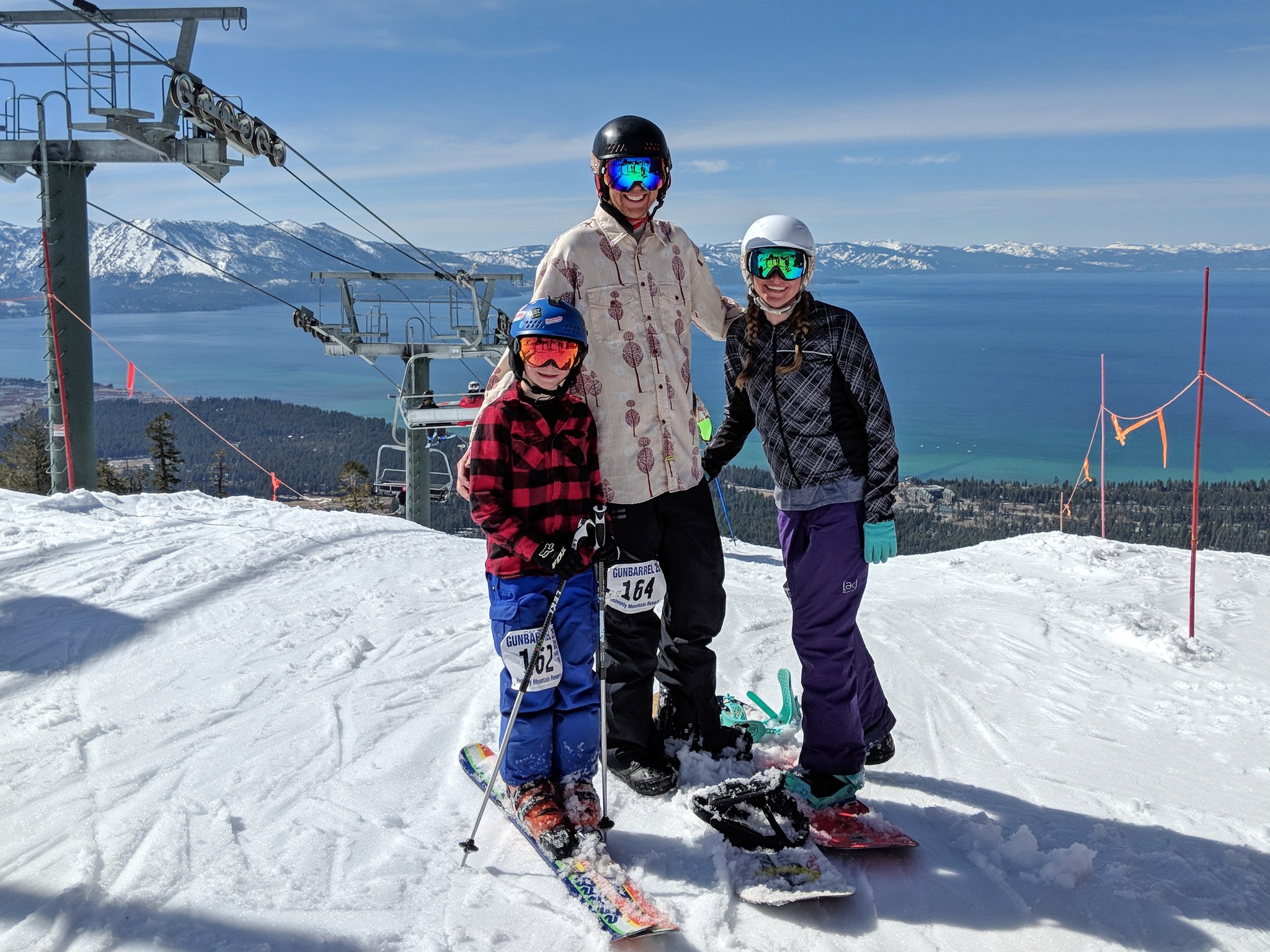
[600,738,677,796]
[865,732,895,765]
[709,764,865,811]
[557,774,601,829]
[654,716,753,761]
[506,775,562,830]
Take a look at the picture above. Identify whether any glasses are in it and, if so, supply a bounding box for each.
[519,336,580,371]
[745,245,808,281]
[603,157,667,190]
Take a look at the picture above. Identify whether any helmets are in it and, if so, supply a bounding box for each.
[740,214,816,291]
[507,297,588,346]
[593,116,670,201]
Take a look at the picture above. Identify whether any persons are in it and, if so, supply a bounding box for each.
[772,258,789,270]
[619,164,645,177]
[466,299,605,840]
[457,381,483,425]
[702,216,898,808]
[546,340,563,352]
[417,390,447,444]
[454,115,752,793]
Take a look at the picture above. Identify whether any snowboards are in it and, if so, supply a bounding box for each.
[752,720,921,852]
[675,692,855,910]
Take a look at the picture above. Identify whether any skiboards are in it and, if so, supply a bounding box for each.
[424,431,457,449]
[460,744,679,941]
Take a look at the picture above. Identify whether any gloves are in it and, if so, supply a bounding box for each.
[531,543,585,581]
[863,520,897,564]
[591,534,620,569]
